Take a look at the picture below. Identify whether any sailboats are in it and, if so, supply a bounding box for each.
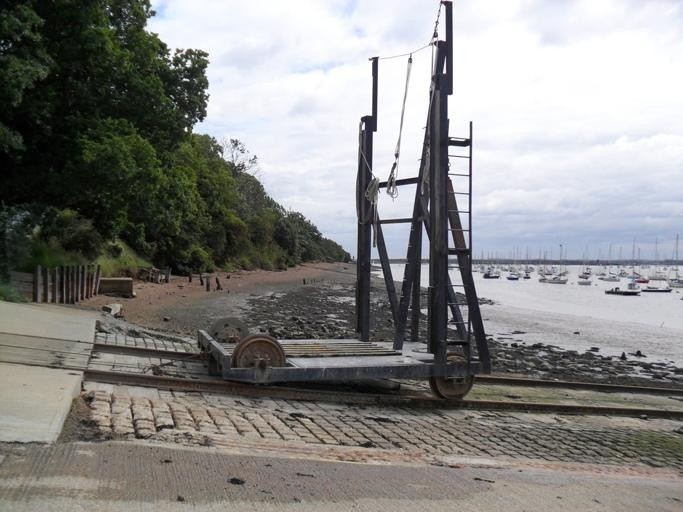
[456,233,683,300]
